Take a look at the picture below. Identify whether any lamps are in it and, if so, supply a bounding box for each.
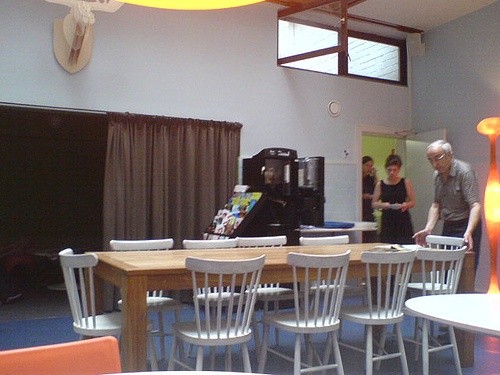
[476,117,500,294]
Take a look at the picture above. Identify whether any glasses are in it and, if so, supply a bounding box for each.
[428,152,447,163]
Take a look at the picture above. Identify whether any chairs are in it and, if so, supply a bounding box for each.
[0,229,466,375]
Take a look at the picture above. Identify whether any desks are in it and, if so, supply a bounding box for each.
[296,222,377,244]
[82,246,476,373]
[406,292,500,335]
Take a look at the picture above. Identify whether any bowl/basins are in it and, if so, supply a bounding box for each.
[389,203,402,210]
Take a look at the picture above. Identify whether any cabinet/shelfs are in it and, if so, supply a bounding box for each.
[241,147,326,310]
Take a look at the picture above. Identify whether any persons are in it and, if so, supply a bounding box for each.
[372,155,416,295]
[412,138,481,347]
[361,156,378,243]
[265,166,274,184]
